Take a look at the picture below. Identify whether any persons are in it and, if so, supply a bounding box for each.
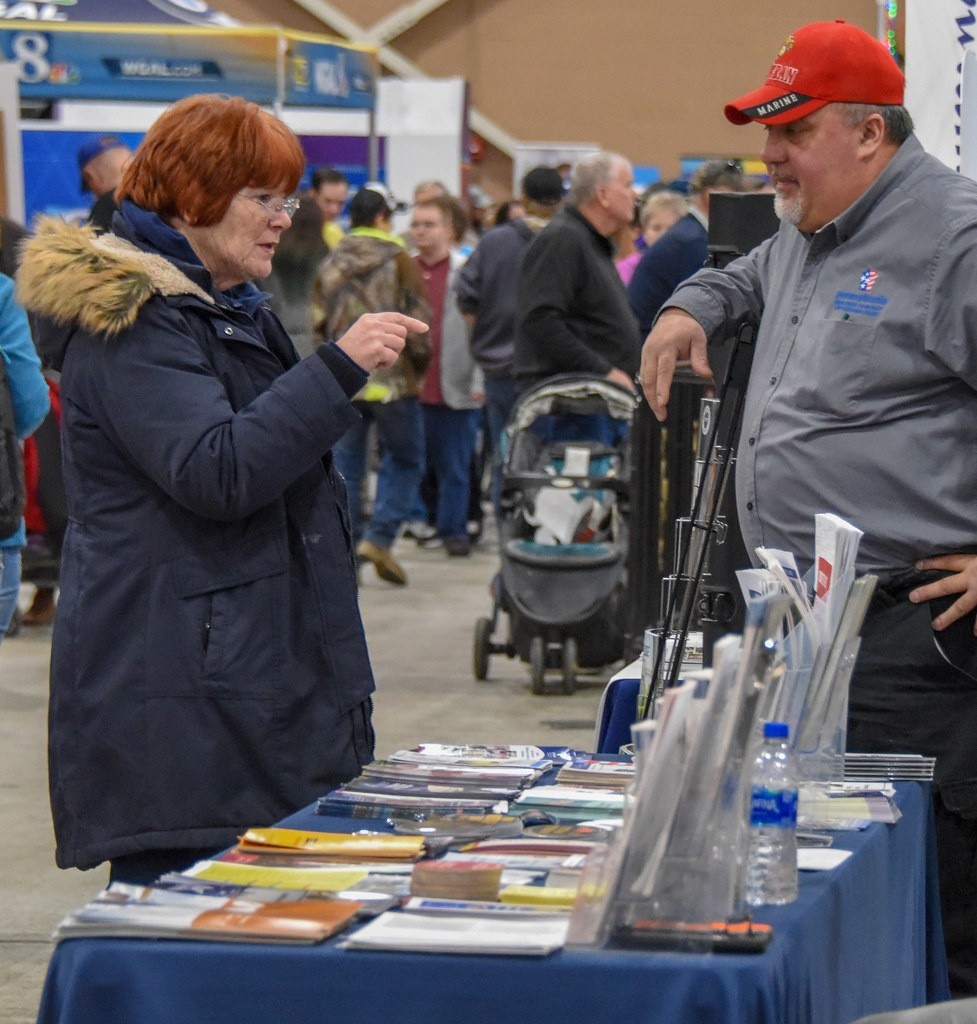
[0,136,767,642]
[16,89,431,885]
[642,19,977,1000]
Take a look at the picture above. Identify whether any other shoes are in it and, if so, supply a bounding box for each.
[446,536,471,556]
[21,597,58,624]
[355,542,407,585]
[464,517,485,542]
[4,606,19,638]
[400,518,437,539]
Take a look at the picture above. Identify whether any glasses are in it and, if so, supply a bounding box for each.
[717,157,744,179]
[235,187,301,222]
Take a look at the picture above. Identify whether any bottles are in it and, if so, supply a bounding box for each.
[741,722,799,907]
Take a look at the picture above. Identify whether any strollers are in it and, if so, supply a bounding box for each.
[473,374,640,696]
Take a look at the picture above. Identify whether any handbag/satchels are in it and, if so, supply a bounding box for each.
[0,356,27,541]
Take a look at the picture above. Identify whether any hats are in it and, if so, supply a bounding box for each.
[724,18,907,126]
[467,185,495,211]
[523,166,563,203]
[366,180,407,211]
[349,187,392,220]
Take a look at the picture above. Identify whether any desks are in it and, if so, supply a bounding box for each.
[593,629,707,757]
[36,746,950,1024]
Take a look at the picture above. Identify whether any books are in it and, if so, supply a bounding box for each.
[593,514,865,950]
[50,742,642,955]
